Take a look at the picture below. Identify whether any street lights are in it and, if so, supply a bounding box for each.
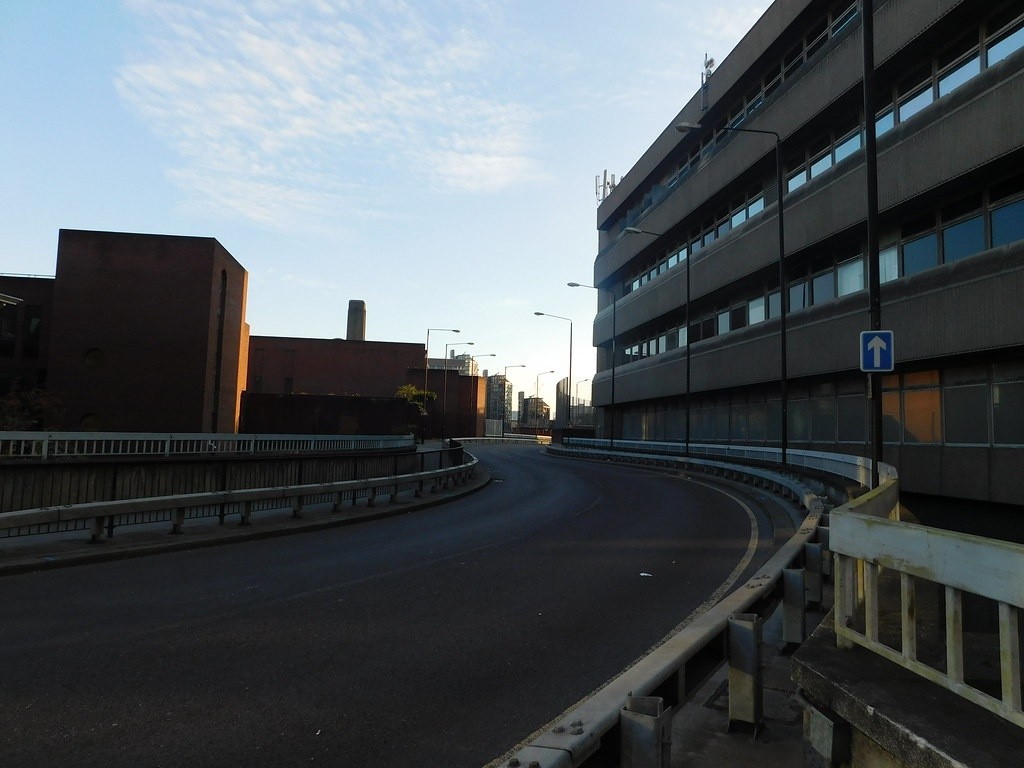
[501,364,526,437]
[439,342,474,442]
[565,282,618,451]
[470,354,497,437]
[421,328,461,444]
[623,225,693,455]
[534,311,573,444]
[675,120,791,480]
[534,370,555,436]
